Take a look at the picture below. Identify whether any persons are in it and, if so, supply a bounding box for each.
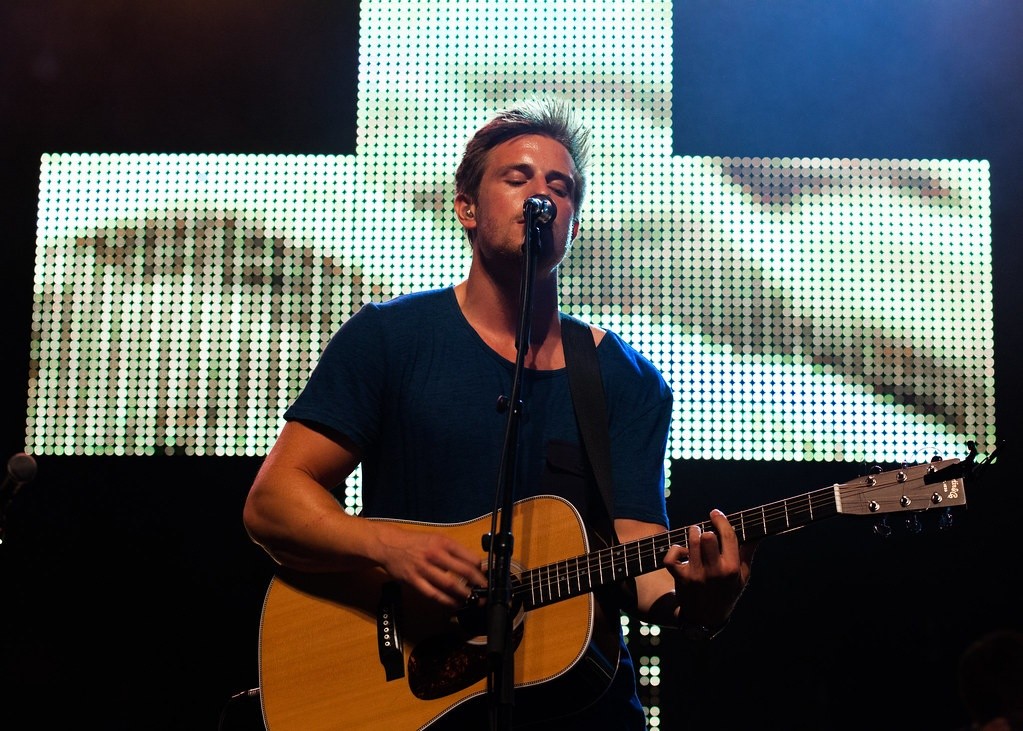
[243,92,754,731]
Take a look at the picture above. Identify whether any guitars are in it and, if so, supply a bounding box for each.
[259,439,1007,731]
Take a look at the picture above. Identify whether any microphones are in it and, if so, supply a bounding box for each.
[523,195,558,227]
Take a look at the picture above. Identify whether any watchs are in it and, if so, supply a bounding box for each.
[679,615,731,642]
